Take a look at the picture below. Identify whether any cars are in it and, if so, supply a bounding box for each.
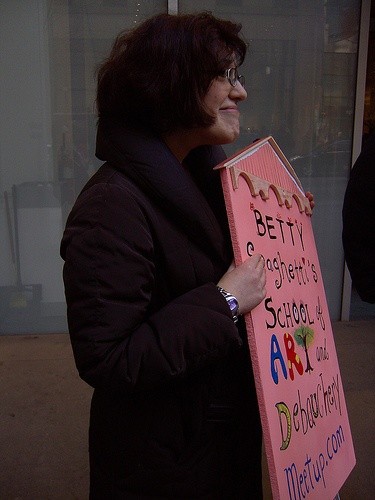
[287,139,353,179]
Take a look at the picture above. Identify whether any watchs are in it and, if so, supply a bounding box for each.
[217,286,239,324]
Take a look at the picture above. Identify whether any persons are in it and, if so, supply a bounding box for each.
[59,11,315,500]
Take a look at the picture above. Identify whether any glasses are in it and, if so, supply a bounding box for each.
[221,68,245,87]
